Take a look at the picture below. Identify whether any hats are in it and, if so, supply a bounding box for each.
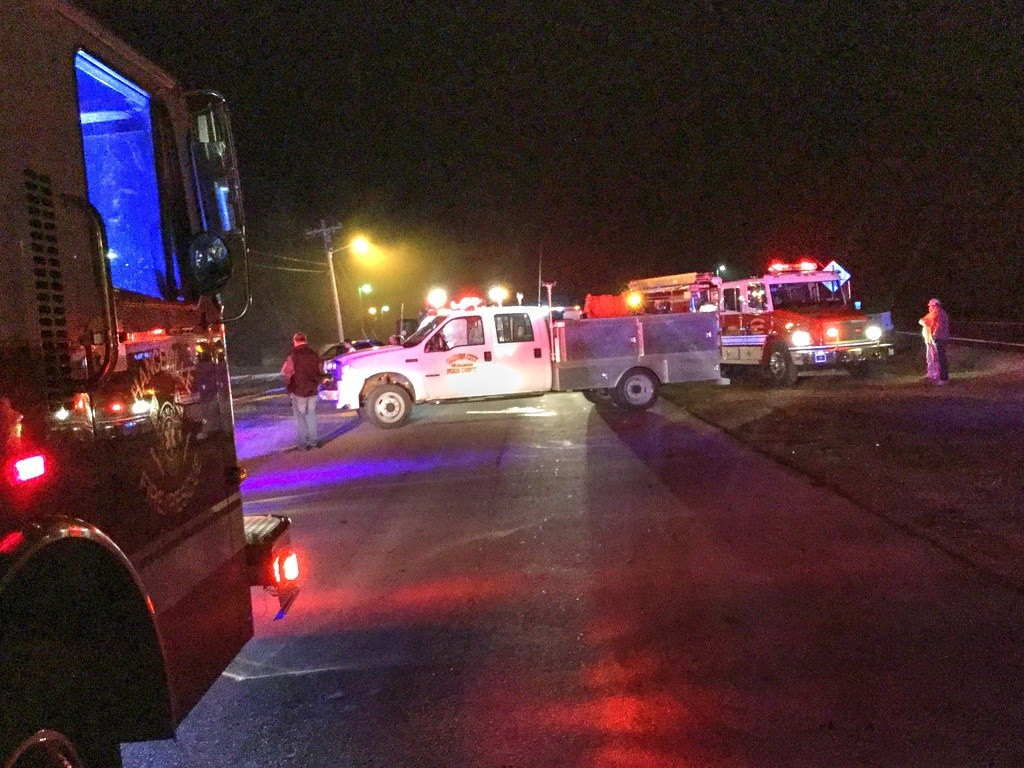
[928,298,940,305]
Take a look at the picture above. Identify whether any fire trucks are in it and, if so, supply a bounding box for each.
[626,258,896,388]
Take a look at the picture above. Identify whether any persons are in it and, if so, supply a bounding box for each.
[280,332,322,452]
[388,335,397,345]
[343,339,356,353]
[918,298,949,385]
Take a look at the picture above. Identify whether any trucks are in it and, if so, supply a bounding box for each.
[0,0,306,768]
[317,304,721,431]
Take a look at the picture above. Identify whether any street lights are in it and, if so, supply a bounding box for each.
[328,234,368,343]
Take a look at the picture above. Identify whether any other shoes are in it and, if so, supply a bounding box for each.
[937,380,949,385]
[297,444,319,451]
